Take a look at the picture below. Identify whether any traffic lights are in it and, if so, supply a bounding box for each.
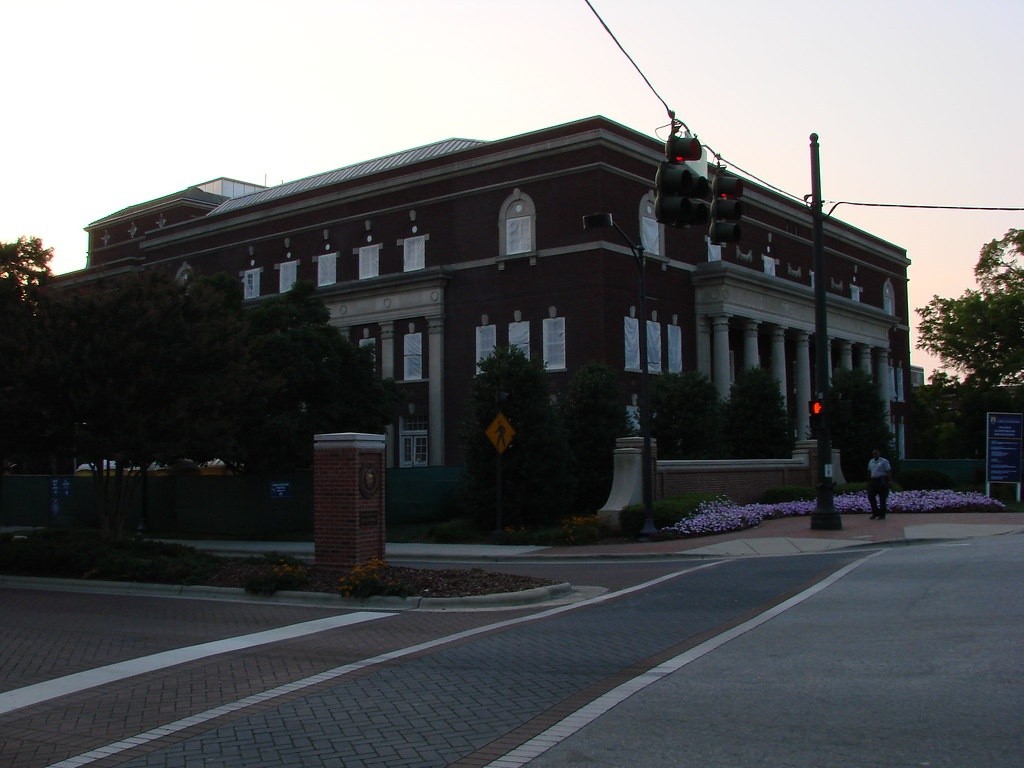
[806,400,826,432]
[654,135,703,226]
[705,171,744,246]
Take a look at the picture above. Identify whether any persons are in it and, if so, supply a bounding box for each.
[867,450,892,519]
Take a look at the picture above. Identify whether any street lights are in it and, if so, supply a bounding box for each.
[585,208,662,547]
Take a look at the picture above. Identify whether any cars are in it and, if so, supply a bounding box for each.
[74,456,232,474]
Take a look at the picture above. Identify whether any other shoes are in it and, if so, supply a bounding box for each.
[870,512,880,519]
[878,514,886,519]
